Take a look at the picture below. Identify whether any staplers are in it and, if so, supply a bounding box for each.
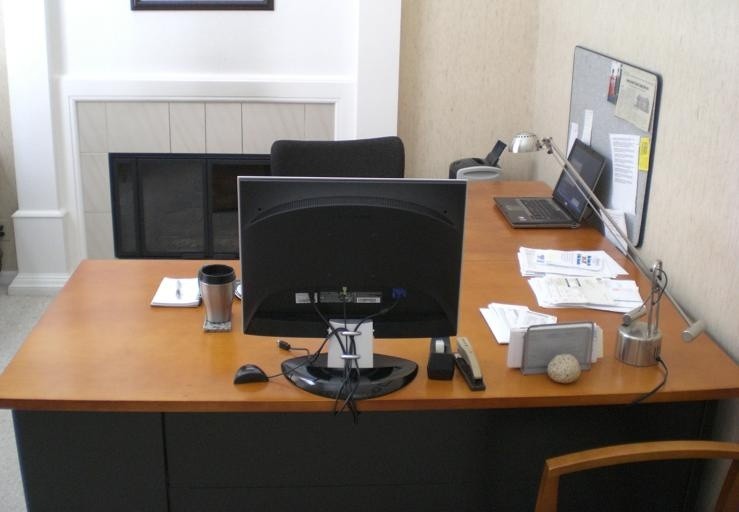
[453,336,487,392]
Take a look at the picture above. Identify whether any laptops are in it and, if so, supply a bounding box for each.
[492,138,606,229]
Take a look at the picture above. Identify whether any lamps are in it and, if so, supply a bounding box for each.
[508,132,706,368]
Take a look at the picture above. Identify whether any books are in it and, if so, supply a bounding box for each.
[150,276,202,308]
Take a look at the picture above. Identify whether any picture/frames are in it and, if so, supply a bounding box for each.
[129,0,275,11]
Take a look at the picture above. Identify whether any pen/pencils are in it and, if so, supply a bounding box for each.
[176,280,181,300]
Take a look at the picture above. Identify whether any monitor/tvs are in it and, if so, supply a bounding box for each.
[237,176,469,398]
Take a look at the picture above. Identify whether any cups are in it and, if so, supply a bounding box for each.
[199,266,236,325]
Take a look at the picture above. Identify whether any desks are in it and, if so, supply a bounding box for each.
[1,182,739,512]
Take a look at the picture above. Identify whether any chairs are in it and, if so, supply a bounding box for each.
[269,134,405,178]
[534,442,739,512]
